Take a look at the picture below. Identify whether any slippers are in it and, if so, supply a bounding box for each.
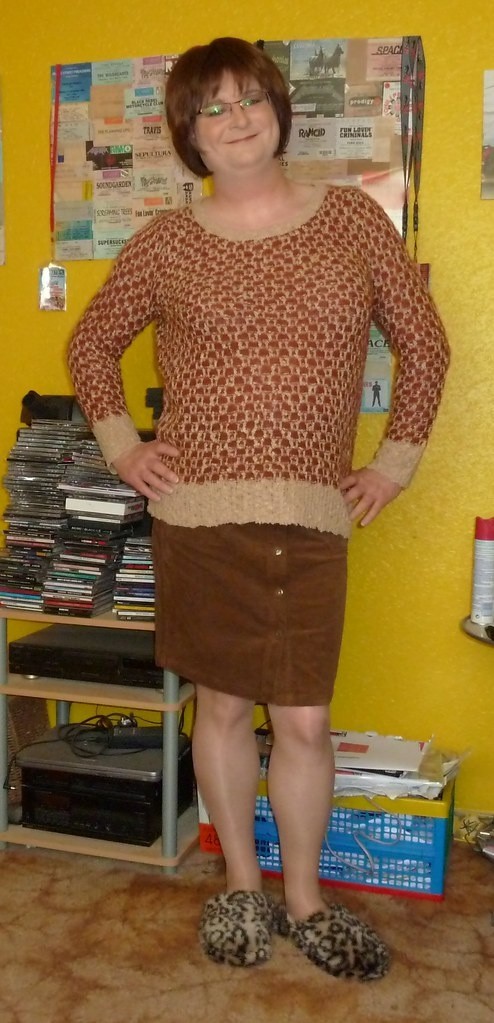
[272,901,389,981]
[199,890,275,967]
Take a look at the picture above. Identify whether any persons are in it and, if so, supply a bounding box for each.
[66,36,449,982]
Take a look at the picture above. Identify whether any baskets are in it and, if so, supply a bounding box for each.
[250,779,457,901]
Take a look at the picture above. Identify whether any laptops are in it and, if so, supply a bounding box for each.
[15,725,190,783]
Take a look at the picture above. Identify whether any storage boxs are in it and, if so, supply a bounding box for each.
[250,771,455,900]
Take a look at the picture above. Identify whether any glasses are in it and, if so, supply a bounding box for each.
[195,92,272,123]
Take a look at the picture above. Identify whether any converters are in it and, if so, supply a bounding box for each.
[107,726,164,749]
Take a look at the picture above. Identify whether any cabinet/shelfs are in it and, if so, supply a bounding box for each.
[0,607,202,875]
